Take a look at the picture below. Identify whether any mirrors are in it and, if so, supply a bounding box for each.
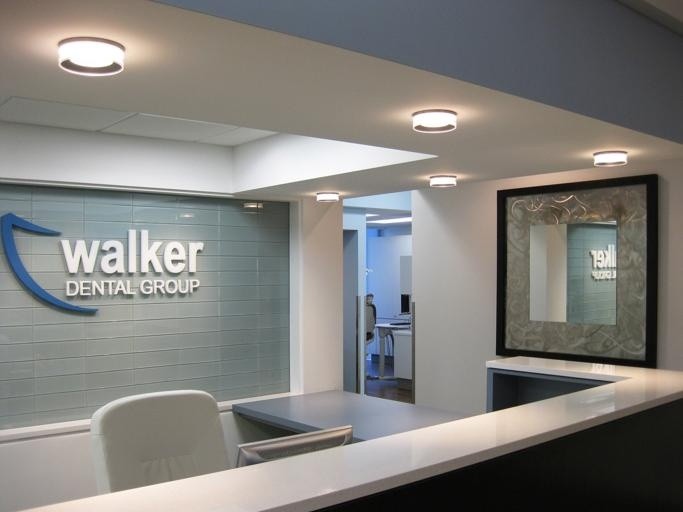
[495,172,658,369]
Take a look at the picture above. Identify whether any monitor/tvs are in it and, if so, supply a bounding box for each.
[235,424,353,468]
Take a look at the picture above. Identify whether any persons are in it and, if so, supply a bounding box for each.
[365,294,376,342]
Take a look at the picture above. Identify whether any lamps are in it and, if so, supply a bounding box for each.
[54,36,126,79]
[315,191,340,204]
[410,108,628,189]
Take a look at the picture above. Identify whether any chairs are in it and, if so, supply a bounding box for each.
[89,388,231,497]
